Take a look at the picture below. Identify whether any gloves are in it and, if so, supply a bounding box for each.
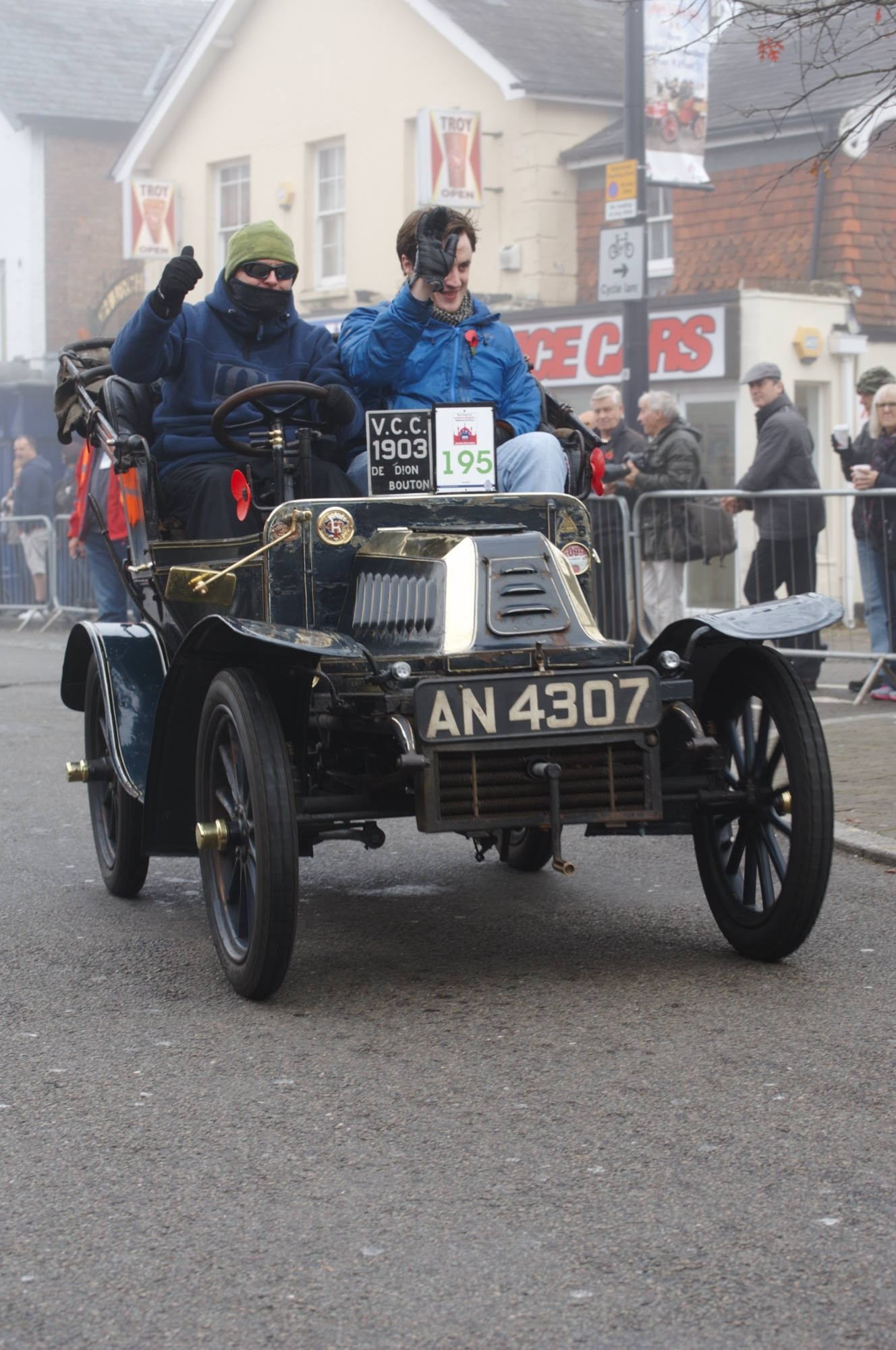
[832,435,853,461]
[410,207,458,294]
[159,246,203,316]
[495,424,510,447]
[318,383,355,425]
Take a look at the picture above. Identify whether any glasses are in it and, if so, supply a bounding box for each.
[242,261,298,281]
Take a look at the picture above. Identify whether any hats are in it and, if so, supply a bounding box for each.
[225,221,299,288]
[740,362,781,384]
[856,365,895,395]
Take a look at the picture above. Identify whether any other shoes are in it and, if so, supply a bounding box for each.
[848,674,883,692]
[17,608,48,623]
[804,678,817,691]
[889,691,896,701]
[870,686,892,700]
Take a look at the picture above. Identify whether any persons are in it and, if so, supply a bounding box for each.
[0,363,896,701]
[118,221,358,637]
[338,205,568,494]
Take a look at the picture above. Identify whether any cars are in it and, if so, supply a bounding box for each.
[54,334,845,998]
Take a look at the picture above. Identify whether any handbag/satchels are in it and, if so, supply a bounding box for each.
[668,500,737,561]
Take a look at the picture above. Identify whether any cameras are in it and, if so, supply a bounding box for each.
[602,450,648,486]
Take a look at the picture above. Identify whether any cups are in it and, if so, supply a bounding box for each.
[143,199,165,242]
[833,426,849,450]
[442,132,468,186]
[852,464,872,489]
